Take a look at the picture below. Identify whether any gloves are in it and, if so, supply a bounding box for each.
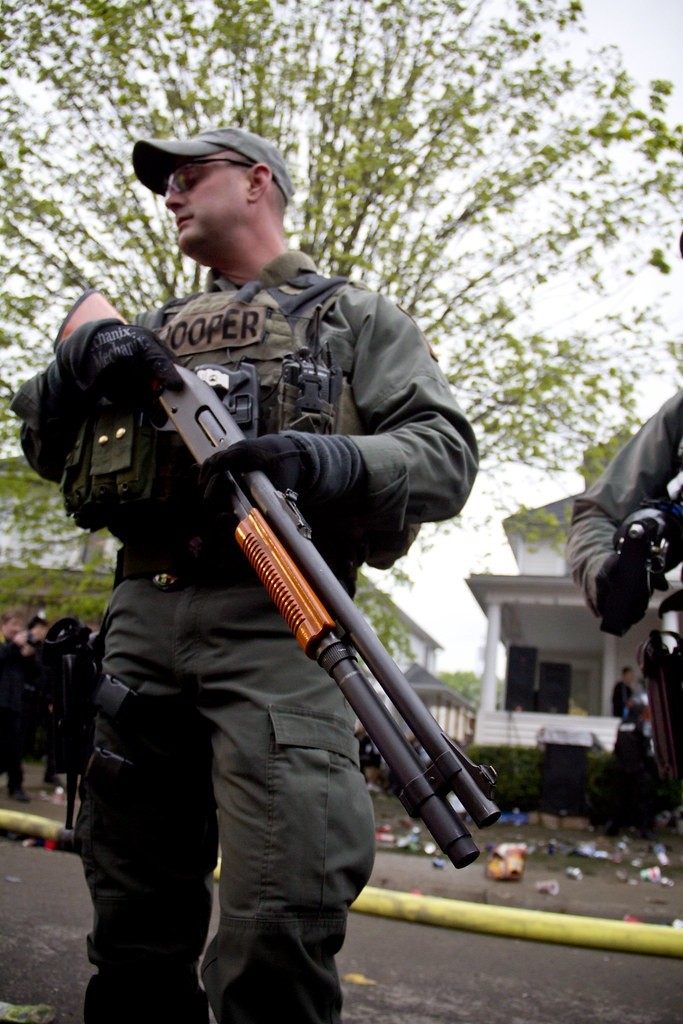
[590,538,655,634]
[89,320,185,413]
[199,434,310,565]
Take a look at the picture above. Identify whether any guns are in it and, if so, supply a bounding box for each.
[50,288,502,872]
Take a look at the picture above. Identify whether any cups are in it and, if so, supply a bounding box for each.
[534,879,560,897]
[640,866,660,880]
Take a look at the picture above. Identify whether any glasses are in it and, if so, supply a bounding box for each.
[165,156,256,192]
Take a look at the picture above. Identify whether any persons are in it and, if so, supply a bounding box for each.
[354,730,383,794]
[571,664,681,835]
[562,387,683,781]
[0,601,101,802]
[13,128,481,1024]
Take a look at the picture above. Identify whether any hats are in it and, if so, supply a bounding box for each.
[130,127,299,203]
